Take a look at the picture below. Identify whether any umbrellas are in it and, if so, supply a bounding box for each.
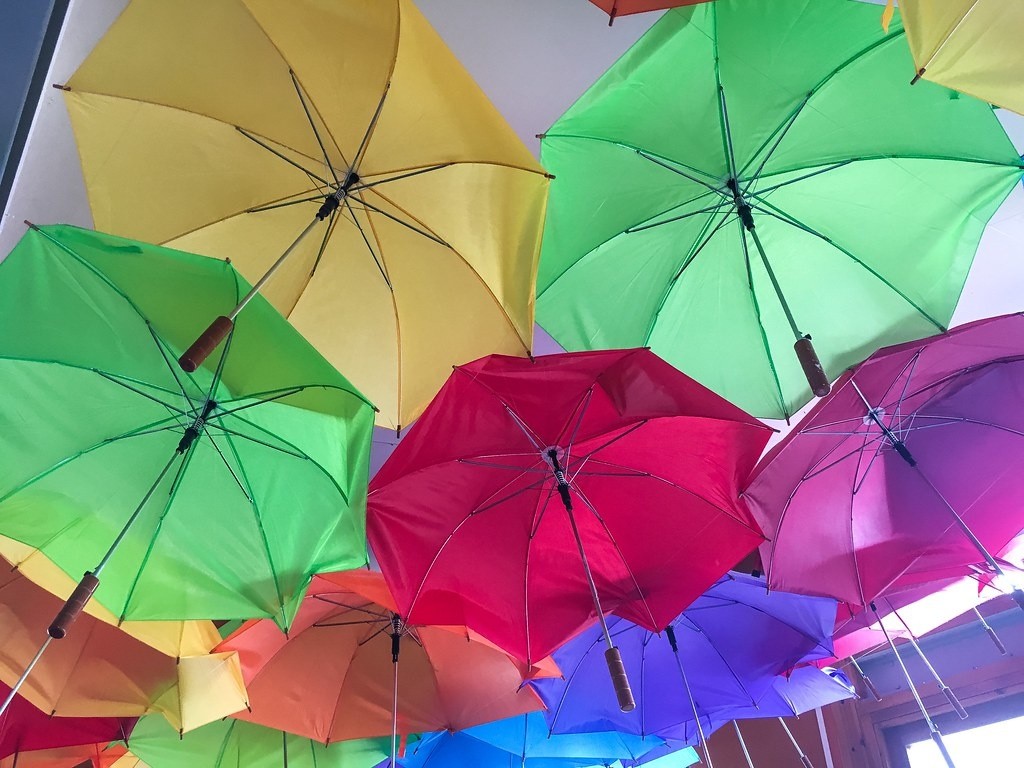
[0,0,1023,768]
